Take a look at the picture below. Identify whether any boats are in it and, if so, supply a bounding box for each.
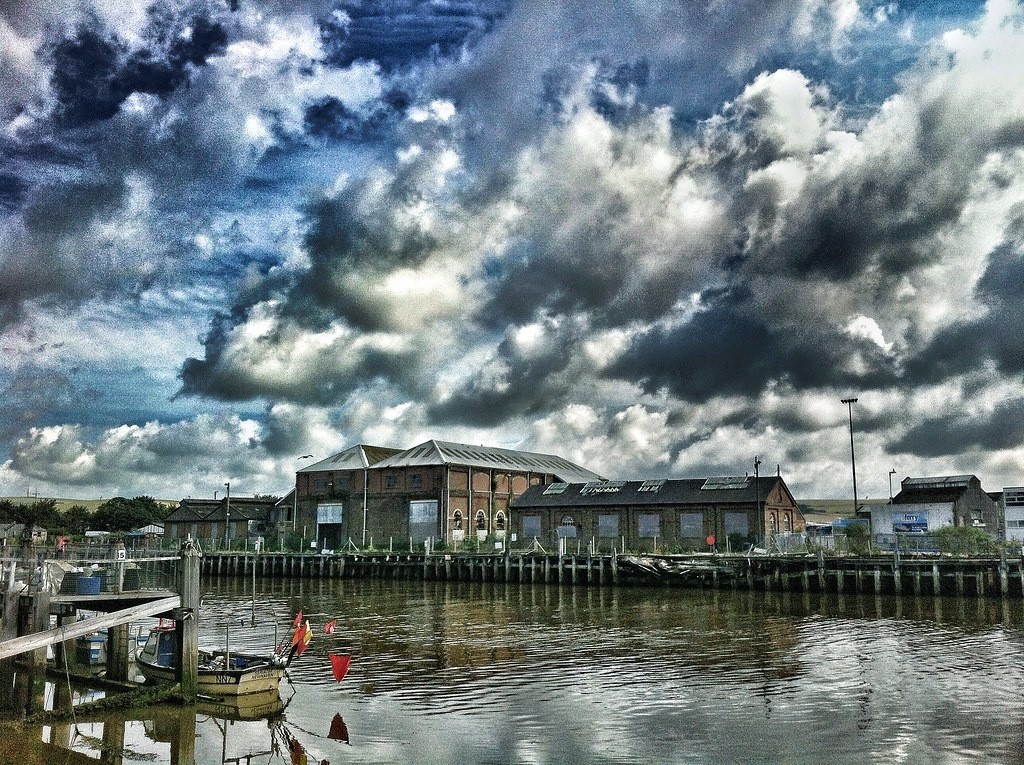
[134,625,278,694]
[193,692,283,720]
[48,609,150,665]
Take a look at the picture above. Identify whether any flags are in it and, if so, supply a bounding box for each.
[292,608,353,682]
[289,712,351,765]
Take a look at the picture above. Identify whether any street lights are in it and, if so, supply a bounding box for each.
[753,455,762,548]
[224,481,230,550]
[840,398,864,519]
[889,468,897,531]
